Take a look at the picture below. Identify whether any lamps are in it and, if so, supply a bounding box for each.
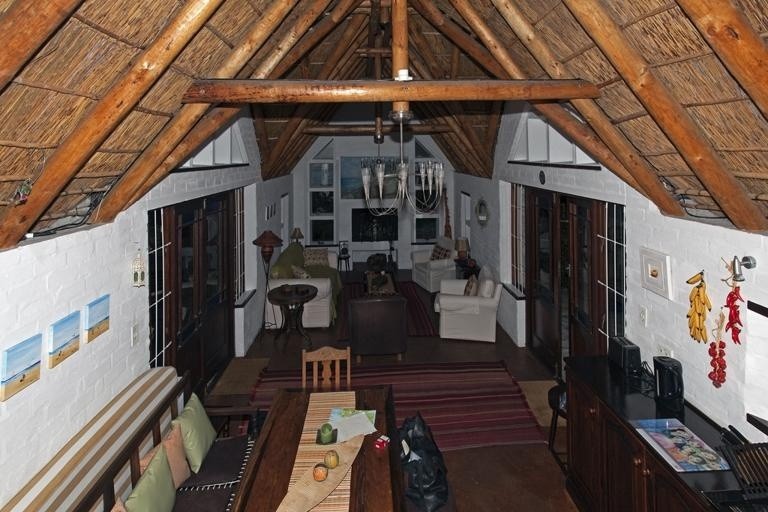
[291,228,304,242]
[252,230,283,336]
[133,249,148,292]
[455,237,470,260]
[361,101,444,217]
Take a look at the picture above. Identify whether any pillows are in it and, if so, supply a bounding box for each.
[430,244,451,261]
[171,393,217,474]
[110,497,126,512]
[291,265,311,279]
[139,422,192,490]
[464,275,477,296]
[123,444,176,512]
[303,248,329,267]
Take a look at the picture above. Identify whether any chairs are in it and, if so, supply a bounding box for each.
[411,239,456,293]
[434,264,503,343]
[302,347,350,388]
[347,296,408,364]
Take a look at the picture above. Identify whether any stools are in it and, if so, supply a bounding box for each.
[338,255,351,272]
[545,384,566,457]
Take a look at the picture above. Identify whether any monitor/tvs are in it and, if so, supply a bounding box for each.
[351,208,398,241]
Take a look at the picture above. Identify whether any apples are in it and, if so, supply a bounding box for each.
[313,464,327,481]
[324,450,338,469]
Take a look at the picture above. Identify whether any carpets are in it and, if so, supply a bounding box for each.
[239,362,548,453]
[336,281,439,341]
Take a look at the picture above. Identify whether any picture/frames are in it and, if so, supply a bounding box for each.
[639,249,668,299]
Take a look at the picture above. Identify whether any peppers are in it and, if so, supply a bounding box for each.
[685,271,712,344]
[725,285,746,344]
[707,340,727,389]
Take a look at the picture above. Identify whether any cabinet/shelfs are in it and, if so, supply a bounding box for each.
[308,159,337,245]
[563,354,768,511]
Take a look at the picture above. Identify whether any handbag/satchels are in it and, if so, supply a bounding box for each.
[396,410,448,512]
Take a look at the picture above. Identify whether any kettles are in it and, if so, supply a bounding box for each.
[651,356,685,412]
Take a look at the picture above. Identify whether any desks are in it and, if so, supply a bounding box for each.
[230,385,399,512]
[268,284,318,347]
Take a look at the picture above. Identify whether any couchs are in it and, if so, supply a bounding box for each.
[72,368,259,512]
[264,242,343,330]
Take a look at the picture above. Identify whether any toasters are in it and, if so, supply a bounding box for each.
[608,333,643,375]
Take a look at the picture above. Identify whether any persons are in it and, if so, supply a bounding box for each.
[648,428,723,471]
